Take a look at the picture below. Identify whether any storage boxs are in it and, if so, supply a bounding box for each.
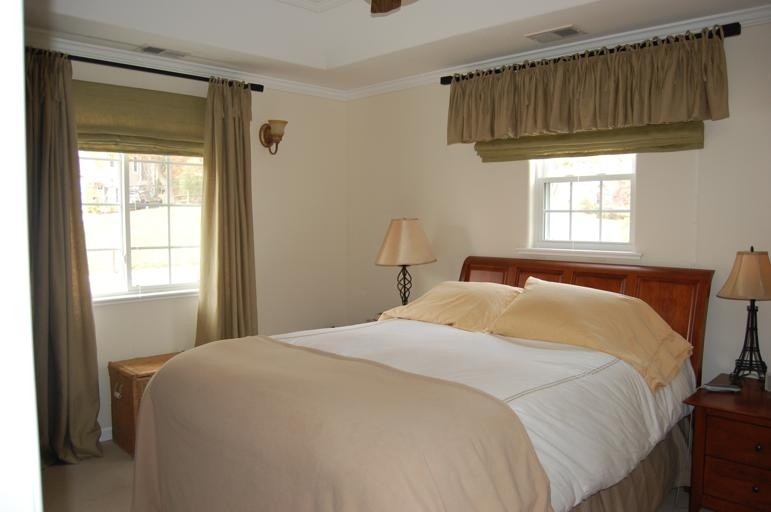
[109,350,187,455]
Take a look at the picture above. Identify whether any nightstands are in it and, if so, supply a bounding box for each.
[682,372,771,511]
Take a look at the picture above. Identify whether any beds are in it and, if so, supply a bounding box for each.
[135,257,715,511]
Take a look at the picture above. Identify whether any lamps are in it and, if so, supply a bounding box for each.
[373,216,439,305]
[716,245,771,388]
[259,119,289,156]
